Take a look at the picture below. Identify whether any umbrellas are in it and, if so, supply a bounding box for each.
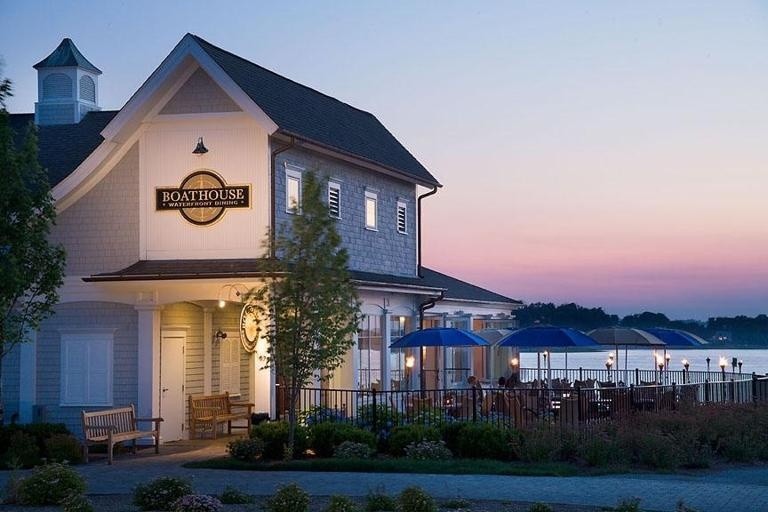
[387,325,491,388]
[469,327,512,346]
[583,326,667,385]
[636,327,710,382]
[491,324,604,396]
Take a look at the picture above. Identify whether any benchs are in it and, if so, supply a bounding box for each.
[187,391,255,440]
[81,401,165,466]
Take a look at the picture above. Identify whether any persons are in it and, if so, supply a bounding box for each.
[510,372,525,387]
[494,377,504,393]
[462,375,479,399]
[502,379,520,397]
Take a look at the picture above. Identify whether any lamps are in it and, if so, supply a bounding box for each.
[191,135,209,156]
[215,330,228,342]
